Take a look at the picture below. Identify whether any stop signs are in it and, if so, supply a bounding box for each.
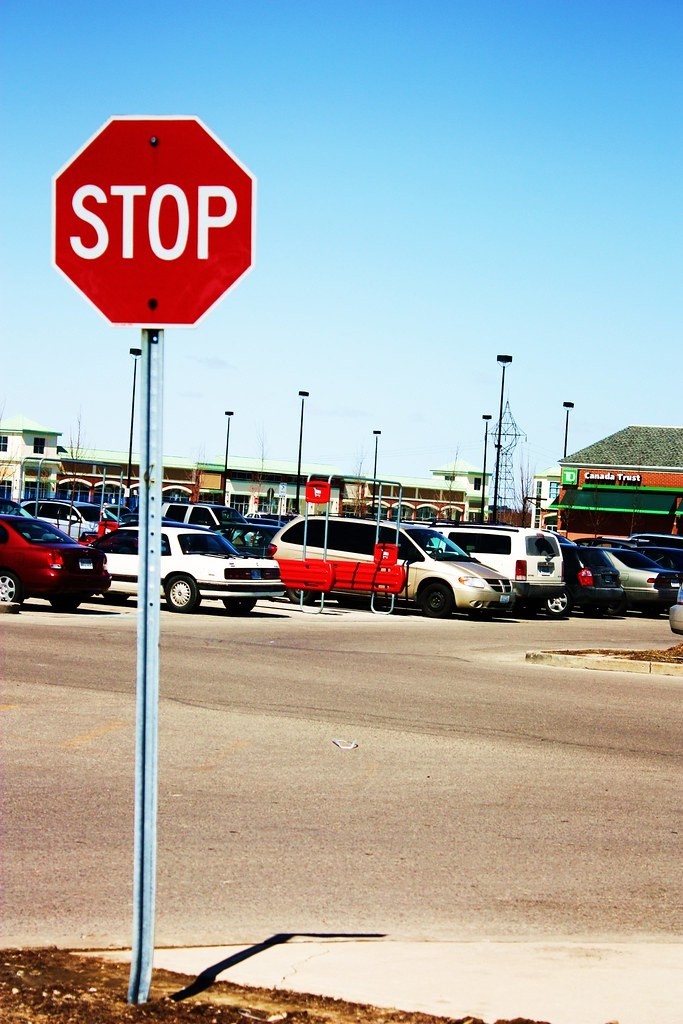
[52,114,258,327]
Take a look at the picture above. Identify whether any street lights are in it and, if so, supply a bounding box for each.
[372,431,381,519]
[494,354,512,520]
[221,410,235,507]
[562,401,575,458]
[124,347,143,488]
[294,391,309,514]
[479,415,493,524]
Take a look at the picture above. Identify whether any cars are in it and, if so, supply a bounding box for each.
[0,514,113,612]
[519,532,624,616]
[0,497,51,538]
[590,546,683,616]
[210,522,286,558]
[89,520,287,615]
[668,582,683,637]
[20,498,122,542]
[571,533,683,571]
[106,505,131,520]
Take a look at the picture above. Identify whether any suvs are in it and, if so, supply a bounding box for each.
[159,500,262,548]
[397,517,565,617]
[263,511,517,619]
